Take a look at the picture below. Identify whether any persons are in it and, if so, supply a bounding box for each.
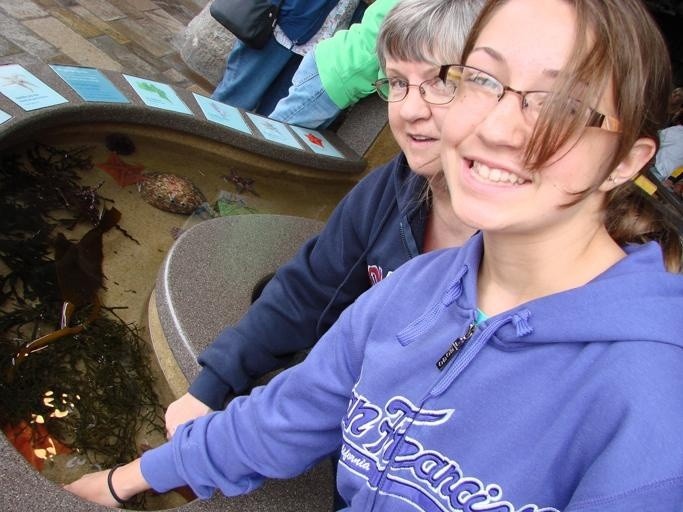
[61,1,683,512]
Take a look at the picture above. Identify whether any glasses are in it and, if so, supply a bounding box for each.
[372,77,458,105]
[438,64,624,134]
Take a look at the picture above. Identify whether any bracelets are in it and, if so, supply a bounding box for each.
[107,462,133,504]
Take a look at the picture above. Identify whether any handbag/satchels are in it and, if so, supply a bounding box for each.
[211,0,279,48]
